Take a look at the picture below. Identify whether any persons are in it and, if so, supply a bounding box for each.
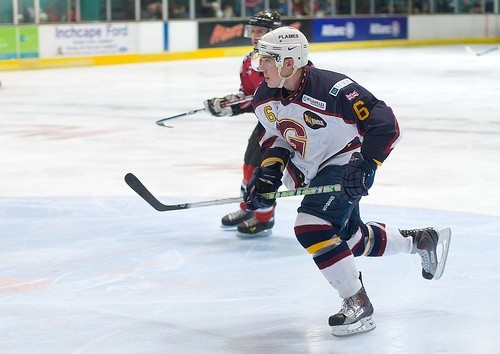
[244,27,452,337]
[16,0,493,18]
[204,9,295,237]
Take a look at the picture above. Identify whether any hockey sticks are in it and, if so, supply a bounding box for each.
[155,94,253,129]
[124,172,341,212]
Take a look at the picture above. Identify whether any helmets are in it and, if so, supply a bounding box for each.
[247,9,283,30]
[254,25,309,80]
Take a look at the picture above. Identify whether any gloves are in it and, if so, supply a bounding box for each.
[339,152,375,206]
[204,94,245,118]
[239,165,284,214]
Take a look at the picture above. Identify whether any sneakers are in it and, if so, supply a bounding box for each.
[219,208,253,230]
[328,271,377,338]
[236,197,276,239]
[397,226,452,280]
